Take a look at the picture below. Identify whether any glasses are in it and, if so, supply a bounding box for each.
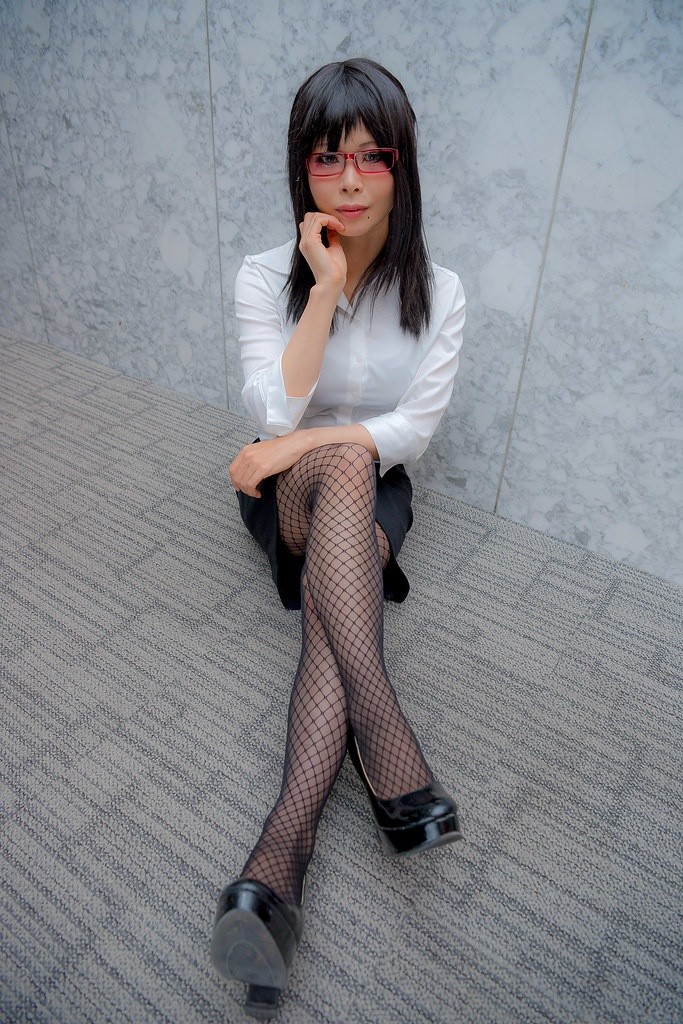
[307,148,399,178]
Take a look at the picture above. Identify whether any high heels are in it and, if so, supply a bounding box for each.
[212,874,305,1019]
[347,718,463,855]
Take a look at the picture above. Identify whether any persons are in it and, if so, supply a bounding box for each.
[209,57,466,1018]
